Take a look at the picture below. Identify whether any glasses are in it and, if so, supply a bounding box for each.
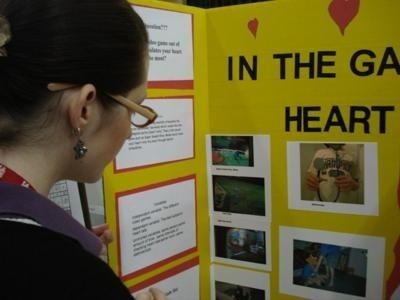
[48,82,157,128]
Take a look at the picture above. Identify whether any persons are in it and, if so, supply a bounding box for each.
[0,1,173,300]
[304,143,360,203]
